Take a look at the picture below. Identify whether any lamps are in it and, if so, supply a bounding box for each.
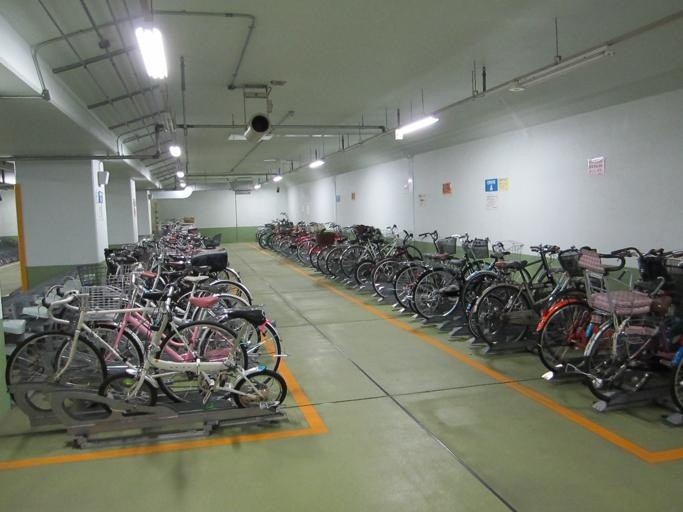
[253,90,439,189]
[135,1,168,80]
[508,45,617,92]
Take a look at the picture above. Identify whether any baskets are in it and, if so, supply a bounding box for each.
[664,255,682,277]
[559,254,583,277]
[462,238,488,259]
[437,237,456,255]
[77,248,138,320]
[502,240,524,262]
[636,256,664,281]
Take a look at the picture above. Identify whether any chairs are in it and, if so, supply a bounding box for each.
[578,249,653,363]
